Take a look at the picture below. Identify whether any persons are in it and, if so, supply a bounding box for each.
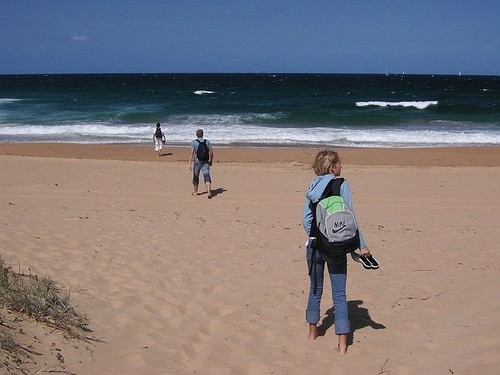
[152,122,166,158]
[189,129,213,199]
[301,148,370,357]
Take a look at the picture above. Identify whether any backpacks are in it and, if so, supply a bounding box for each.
[196,140,209,161]
[314,179,360,257]
[156,128,162,138]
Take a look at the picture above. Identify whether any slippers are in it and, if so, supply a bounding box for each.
[353,253,379,270]
